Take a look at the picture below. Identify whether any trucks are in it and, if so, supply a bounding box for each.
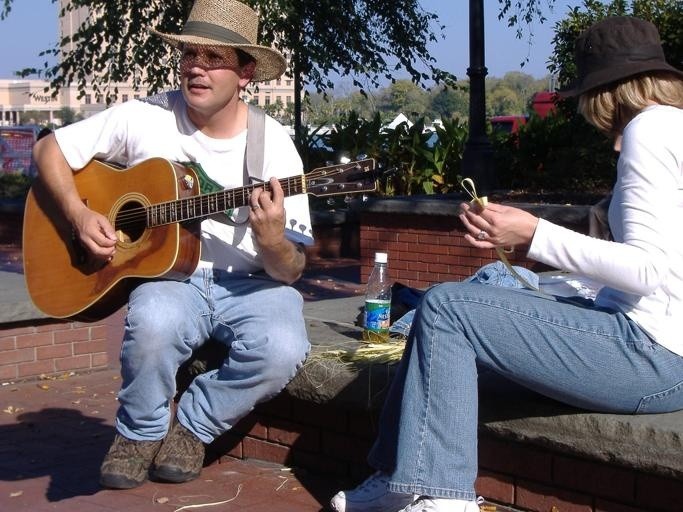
[487,93,565,151]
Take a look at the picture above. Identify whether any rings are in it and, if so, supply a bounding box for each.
[252,203,260,210]
[478,230,484,240]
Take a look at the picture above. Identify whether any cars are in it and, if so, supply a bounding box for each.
[0,124,43,214]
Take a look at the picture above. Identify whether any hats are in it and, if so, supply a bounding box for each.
[557,17,682,97]
[147,0,287,81]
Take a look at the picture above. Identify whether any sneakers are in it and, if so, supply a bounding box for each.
[149,412,205,483]
[398,496,485,512]
[330,471,414,512]
[98,433,163,489]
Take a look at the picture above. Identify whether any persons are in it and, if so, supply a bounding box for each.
[30,1,315,487]
[329,19,683,510]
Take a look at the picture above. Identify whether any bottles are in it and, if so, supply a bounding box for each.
[365,252,390,346]
